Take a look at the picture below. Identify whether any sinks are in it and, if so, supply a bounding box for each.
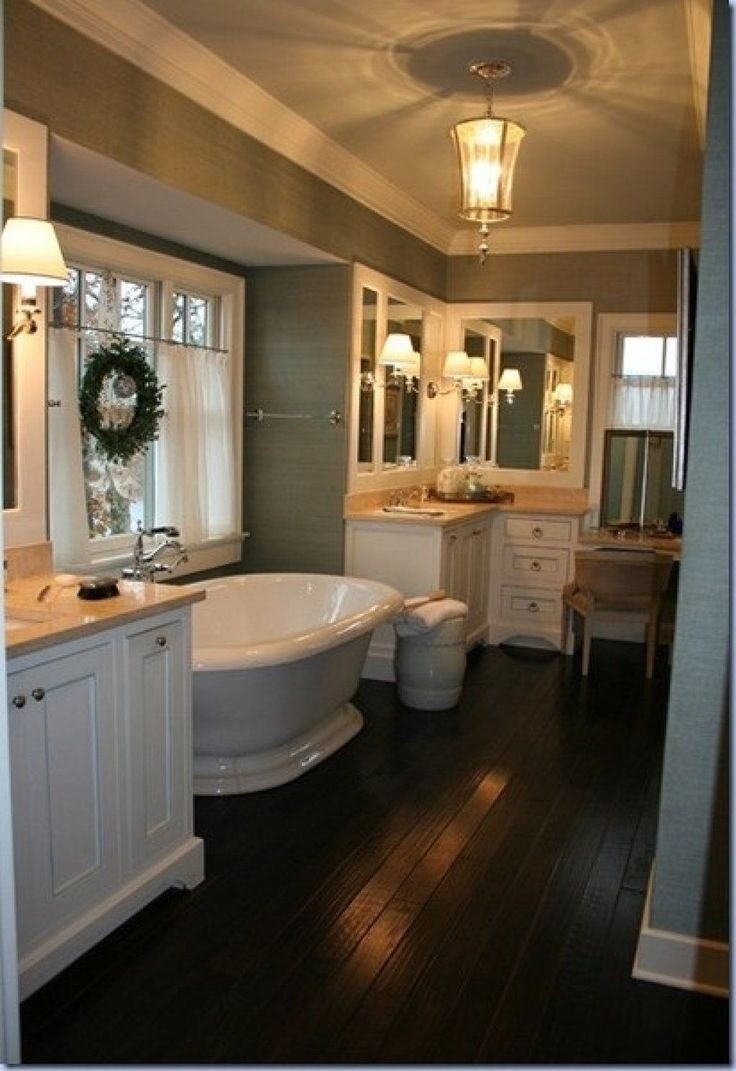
[377,506,444,518]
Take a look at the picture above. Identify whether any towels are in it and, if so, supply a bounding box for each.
[385,594,469,639]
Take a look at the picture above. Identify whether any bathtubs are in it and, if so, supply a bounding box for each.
[179,568,405,795]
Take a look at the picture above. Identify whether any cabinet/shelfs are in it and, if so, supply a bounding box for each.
[343,515,591,683]
[5,607,204,1004]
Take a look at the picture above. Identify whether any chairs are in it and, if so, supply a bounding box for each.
[558,544,671,678]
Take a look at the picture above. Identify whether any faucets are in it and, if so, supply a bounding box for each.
[389,487,419,506]
[132,519,189,583]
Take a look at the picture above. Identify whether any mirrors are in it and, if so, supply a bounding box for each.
[457,314,577,471]
[381,295,423,470]
[354,287,382,473]
[598,428,683,534]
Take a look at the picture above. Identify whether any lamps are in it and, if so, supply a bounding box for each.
[0,216,72,340]
[379,333,414,376]
[462,358,490,402]
[497,369,523,403]
[398,350,421,394]
[553,382,573,417]
[428,351,466,398]
[448,57,529,268]
